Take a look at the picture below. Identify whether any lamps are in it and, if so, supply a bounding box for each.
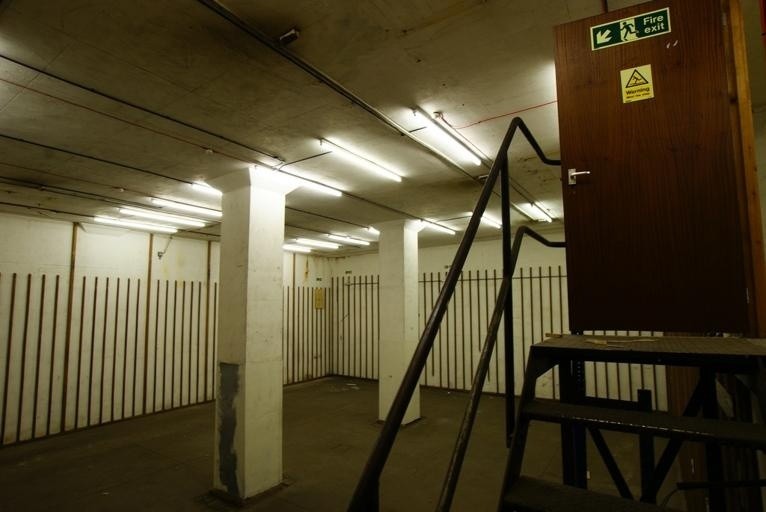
[89,106,554,256]
